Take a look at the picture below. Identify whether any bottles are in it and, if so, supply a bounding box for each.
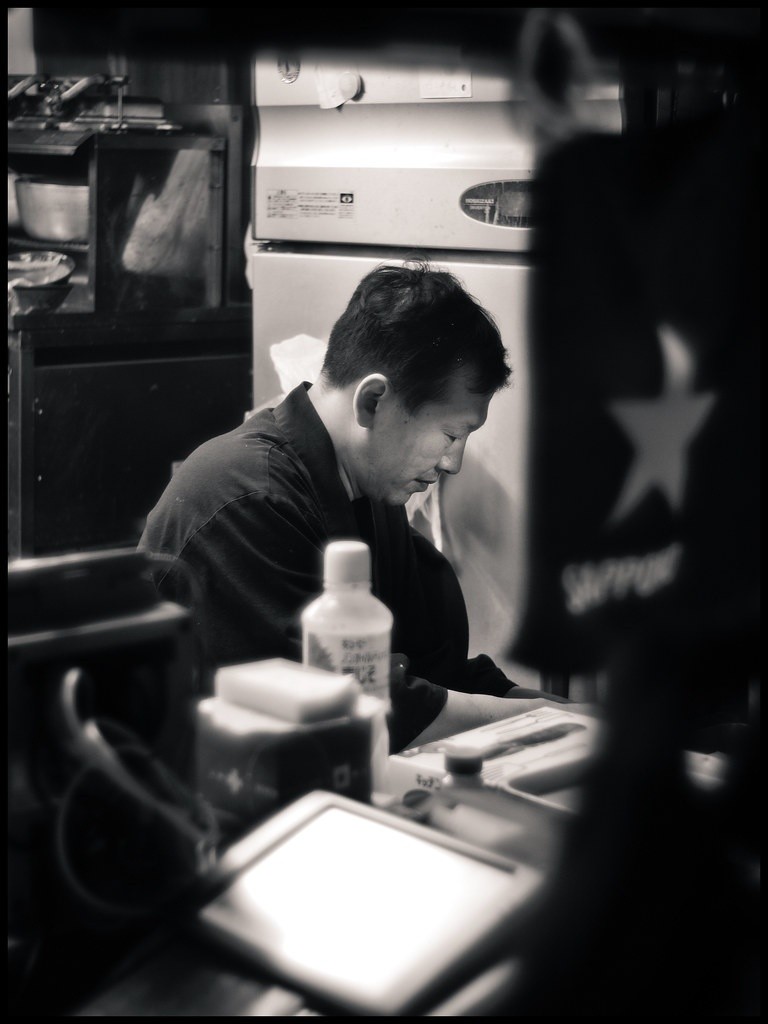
[297,537,395,714]
[437,749,485,789]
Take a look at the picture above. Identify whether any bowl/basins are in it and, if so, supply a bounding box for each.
[14,177,88,242]
[7,250,77,309]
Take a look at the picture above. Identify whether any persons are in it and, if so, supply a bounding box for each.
[132,266,611,759]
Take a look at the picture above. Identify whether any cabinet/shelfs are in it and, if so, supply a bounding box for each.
[6,298,251,554]
